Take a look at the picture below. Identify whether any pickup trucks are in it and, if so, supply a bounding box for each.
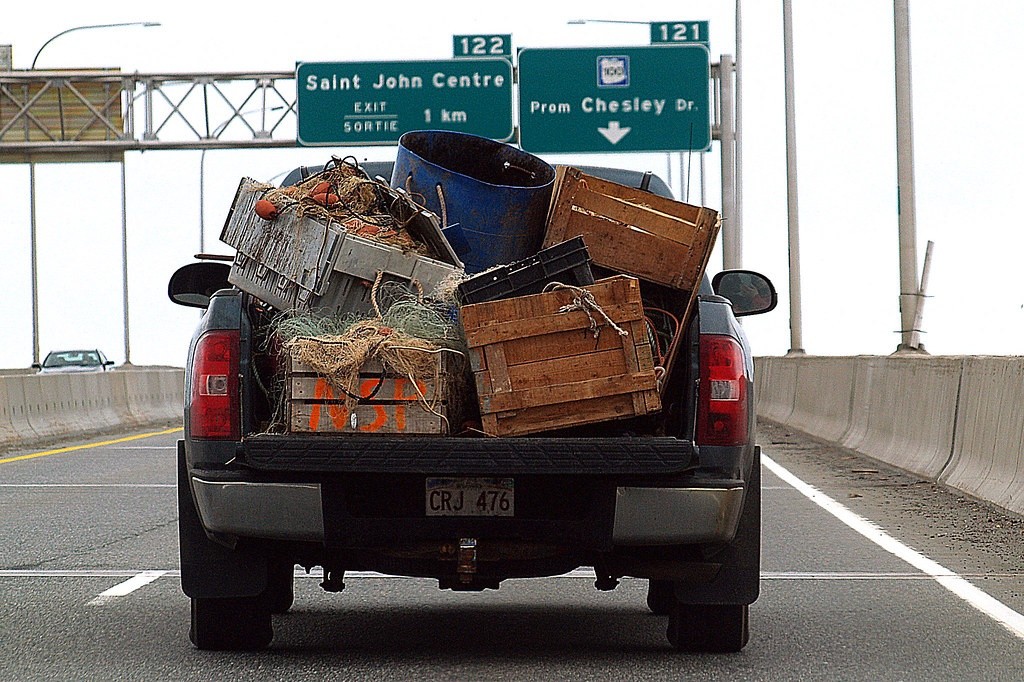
[168,161,780,654]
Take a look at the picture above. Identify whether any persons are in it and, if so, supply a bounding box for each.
[735,276,771,311]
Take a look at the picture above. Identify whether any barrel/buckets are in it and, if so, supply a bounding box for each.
[390,128,554,273]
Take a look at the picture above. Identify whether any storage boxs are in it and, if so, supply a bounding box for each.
[460,273,662,436]
[539,164,720,297]
[285,351,452,438]
[453,232,595,304]
[220,175,464,324]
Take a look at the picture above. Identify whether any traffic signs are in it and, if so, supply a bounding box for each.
[296,56,514,148]
[518,44,713,155]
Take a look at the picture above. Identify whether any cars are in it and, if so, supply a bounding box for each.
[31,350,115,375]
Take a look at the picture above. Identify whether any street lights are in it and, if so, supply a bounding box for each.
[200,106,285,263]
[29,21,162,365]
[567,19,705,207]
